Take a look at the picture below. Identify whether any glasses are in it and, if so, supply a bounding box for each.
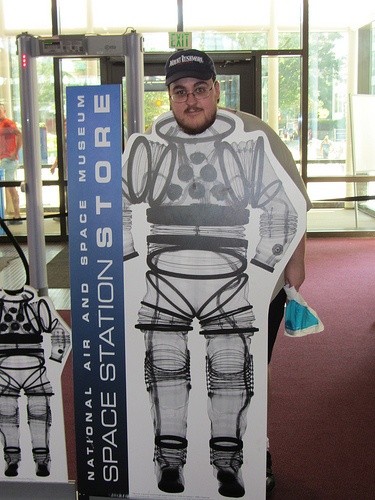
[170,85,215,102]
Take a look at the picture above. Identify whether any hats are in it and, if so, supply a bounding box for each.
[164,47,217,86]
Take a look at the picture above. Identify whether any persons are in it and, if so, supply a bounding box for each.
[320,135,332,159]
[165,48,313,366]
[0,104,24,226]
[50,119,68,212]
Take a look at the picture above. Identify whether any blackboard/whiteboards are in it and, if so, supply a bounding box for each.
[357,24,372,95]
